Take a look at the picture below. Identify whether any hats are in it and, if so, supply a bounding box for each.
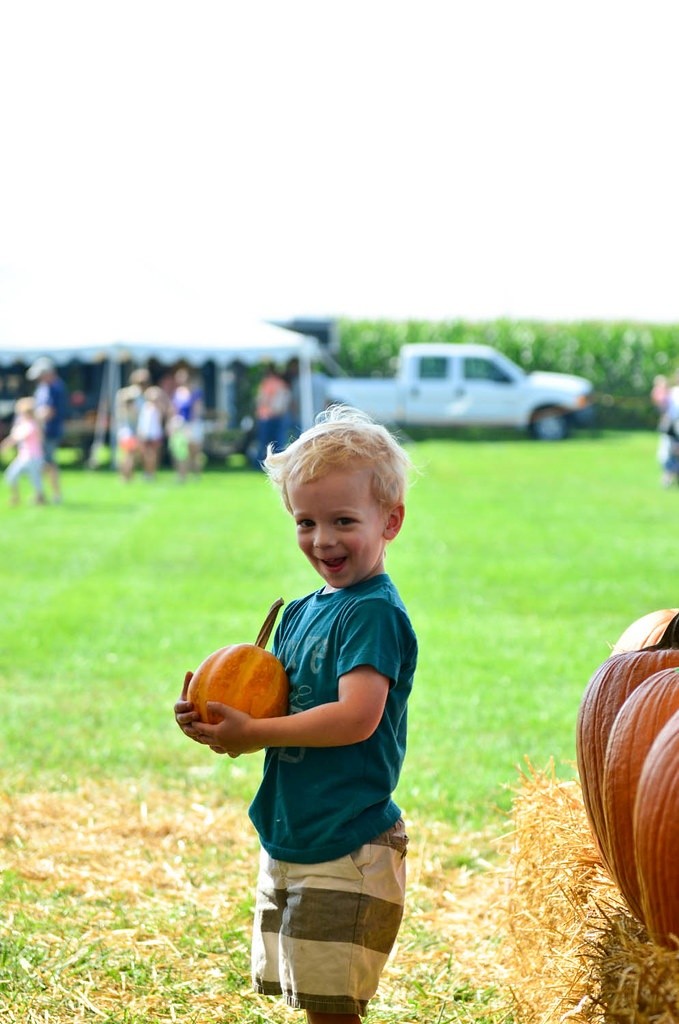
[25,357,52,380]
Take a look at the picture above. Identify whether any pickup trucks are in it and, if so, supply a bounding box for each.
[325,342,598,439]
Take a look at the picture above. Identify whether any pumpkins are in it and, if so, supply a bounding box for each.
[578,607,679,955]
[184,644,289,725]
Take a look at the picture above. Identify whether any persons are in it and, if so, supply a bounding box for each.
[1,357,67,506]
[651,375,679,488]
[115,364,205,486]
[255,359,303,471]
[174,405,418,1024]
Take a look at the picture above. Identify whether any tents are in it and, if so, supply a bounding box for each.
[0,319,320,432]
